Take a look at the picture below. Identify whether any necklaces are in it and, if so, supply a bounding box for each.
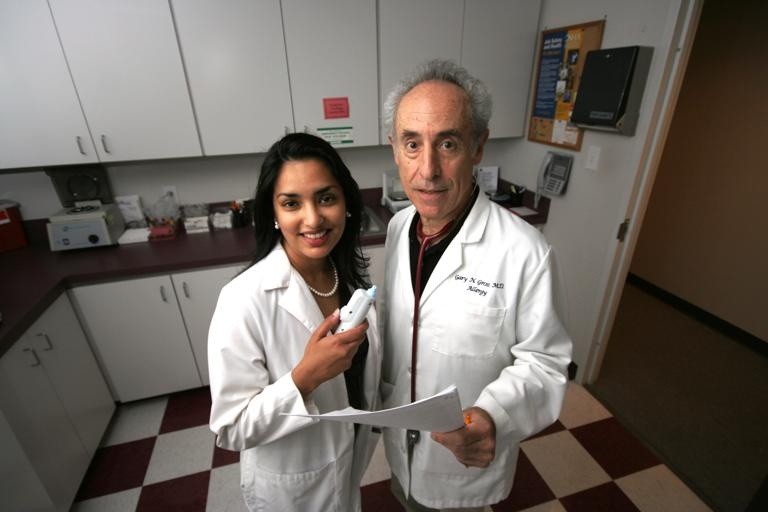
[304,254,343,298]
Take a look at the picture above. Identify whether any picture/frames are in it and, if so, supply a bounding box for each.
[528,19,606,152]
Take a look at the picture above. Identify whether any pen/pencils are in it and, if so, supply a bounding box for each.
[510,185,525,194]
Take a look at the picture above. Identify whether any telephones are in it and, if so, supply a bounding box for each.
[536,150,573,196]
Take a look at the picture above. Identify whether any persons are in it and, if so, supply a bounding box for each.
[378,59,575,511]
[207,132,386,511]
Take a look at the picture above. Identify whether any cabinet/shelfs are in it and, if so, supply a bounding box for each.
[0,410,57,512]
[0,1,203,168]
[0,291,117,511]
[169,1,379,157]
[379,0,542,145]
[363,244,386,302]
[67,262,247,405]
